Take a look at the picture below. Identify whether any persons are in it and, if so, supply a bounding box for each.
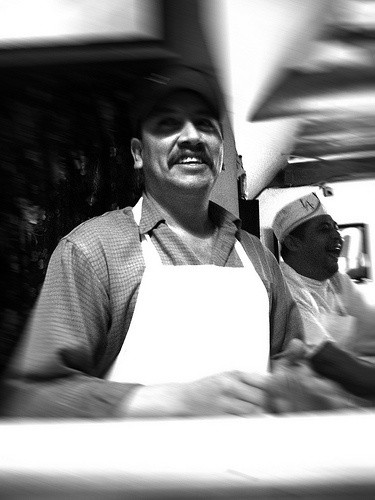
[3,63,316,418]
[270,192,375,410]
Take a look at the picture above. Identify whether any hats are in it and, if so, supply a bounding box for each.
[272,192,333,243]
[131,64,221,135]
